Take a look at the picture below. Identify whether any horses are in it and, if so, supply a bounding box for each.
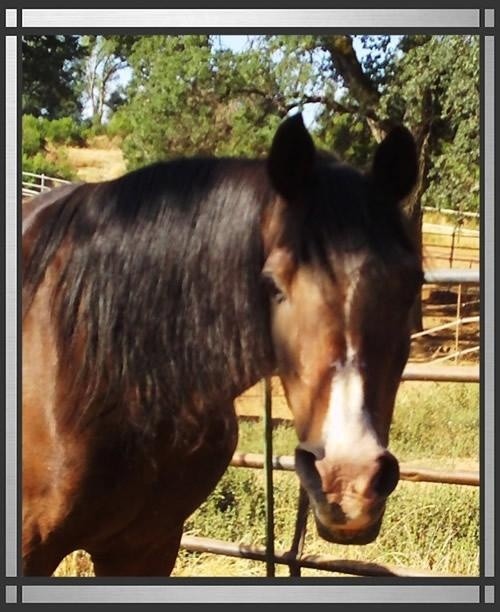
[22,111,425,580]
